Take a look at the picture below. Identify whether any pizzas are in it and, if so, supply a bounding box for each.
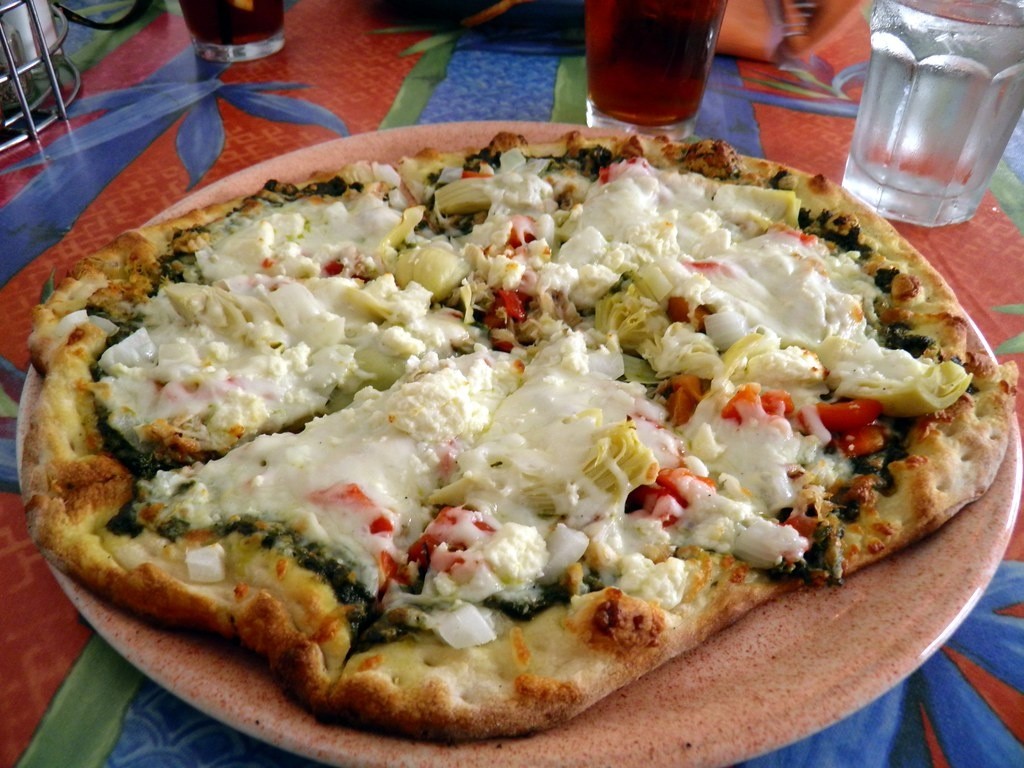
[25,127,1023,743]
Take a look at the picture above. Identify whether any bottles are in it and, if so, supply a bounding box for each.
[0,18,29,112]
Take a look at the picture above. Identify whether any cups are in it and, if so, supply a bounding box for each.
[177,0,288,62]
[0,0,61,76]
[579,0,726,142]
[841,0,1024,227]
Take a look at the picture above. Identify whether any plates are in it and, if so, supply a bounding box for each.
[15,120,1024,768]
